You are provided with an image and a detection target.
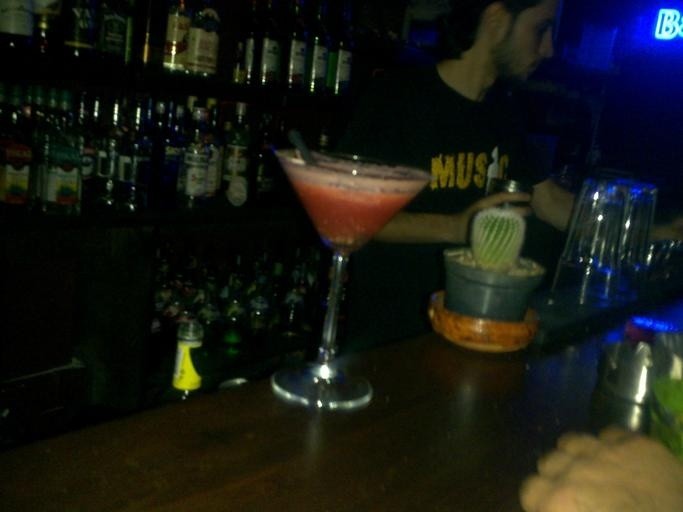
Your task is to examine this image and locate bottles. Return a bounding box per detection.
[158,3,354,97]
[155,247,353,398]
[0,1,151,68]
[2,85,352,219]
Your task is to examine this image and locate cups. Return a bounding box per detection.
[620,180,659,270]
[561,178,624,271]
[487,177,536,214]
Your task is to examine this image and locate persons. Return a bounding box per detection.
[514,422,682,511]
[340,0,682,358]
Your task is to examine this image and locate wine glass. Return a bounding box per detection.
[268,148,431,412]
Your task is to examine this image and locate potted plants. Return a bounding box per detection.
[444,209,546,323]
